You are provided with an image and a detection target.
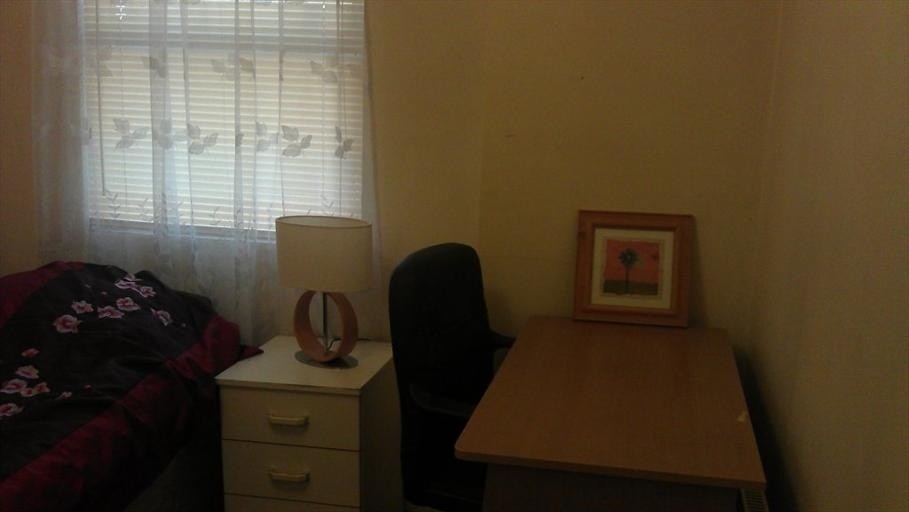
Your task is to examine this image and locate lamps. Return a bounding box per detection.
[276,215,372,363]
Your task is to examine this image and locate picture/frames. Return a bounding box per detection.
[575,210,694,327]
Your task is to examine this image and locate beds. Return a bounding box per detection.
[2,261,223,510]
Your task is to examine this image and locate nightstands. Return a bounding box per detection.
[216,335,403,511]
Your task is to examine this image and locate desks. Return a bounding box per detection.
[455,319,767,512]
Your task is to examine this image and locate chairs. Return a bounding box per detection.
[389,244,519,512]
